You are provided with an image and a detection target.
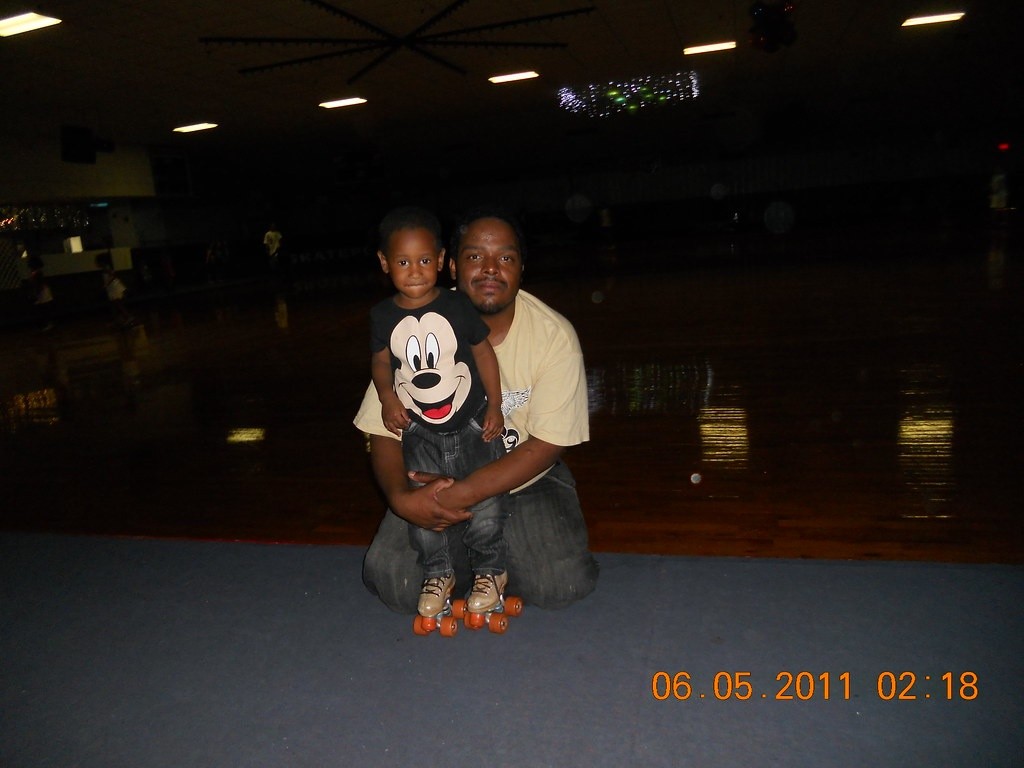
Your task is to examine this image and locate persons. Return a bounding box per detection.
[205,242,226,283]
[263,222,283,255]
[15,242,178,332]
[352,211,600,614]
[598,202,616,251]
[369,207,523,636]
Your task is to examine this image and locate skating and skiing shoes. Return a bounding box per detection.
[464,568,523,634]
[413,571,467,638]
